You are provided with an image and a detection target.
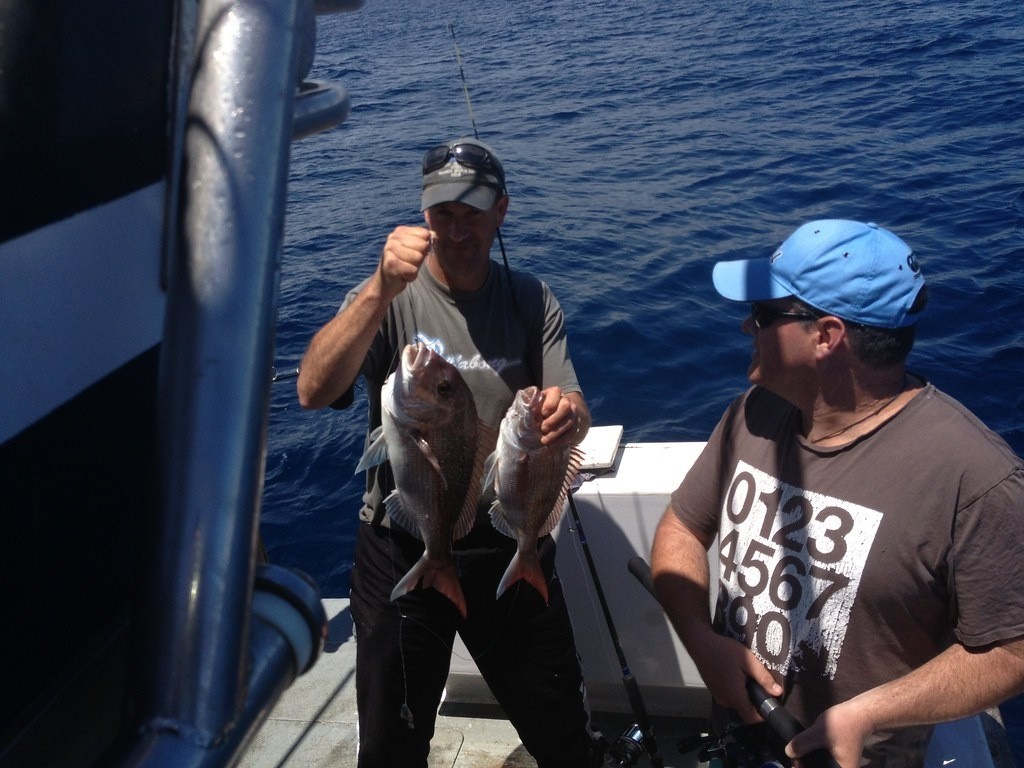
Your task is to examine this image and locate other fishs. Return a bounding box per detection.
[355,341,499,619]
[481,387,587,606]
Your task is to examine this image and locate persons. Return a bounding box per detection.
[647,217,1024,768]
[293,135,615,768]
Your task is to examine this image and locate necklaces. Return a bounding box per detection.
[810,375,907,446]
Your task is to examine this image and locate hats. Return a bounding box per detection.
[421,146,505,214]
[712,218,931,330]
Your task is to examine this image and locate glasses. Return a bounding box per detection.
[422,141,505,184]
[743,301,819,332]
